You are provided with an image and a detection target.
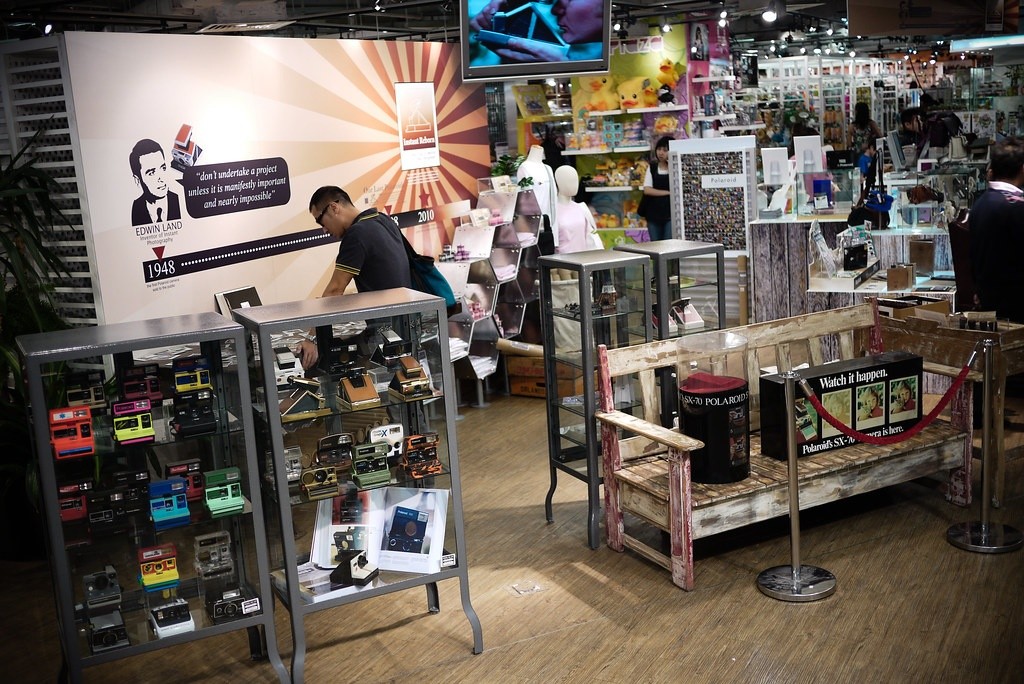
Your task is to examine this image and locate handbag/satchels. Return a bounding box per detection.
[398,226,456,308]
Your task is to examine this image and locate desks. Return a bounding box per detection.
[131,320,363,372]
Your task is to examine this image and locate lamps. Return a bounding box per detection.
[660,13,672,33]
[782,12,965,66]
[719,0,730,29]
[761,0,777,23]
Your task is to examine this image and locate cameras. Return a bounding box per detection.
[46,329,443,653]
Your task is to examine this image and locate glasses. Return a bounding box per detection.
[315,200,339,228]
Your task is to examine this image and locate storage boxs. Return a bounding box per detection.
[505,351,598,399]
[887,263,916,291]
[758,349,923,461]
[603,117,642,149]
[808,259,881,291]
[952,110,996,143]
[878,295,997,329]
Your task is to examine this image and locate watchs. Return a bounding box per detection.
[305,335,317,345]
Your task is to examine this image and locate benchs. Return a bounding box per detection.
[597,303,995,592]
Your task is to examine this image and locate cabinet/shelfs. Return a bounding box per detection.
[691,54,936,148]
[538,249,653,552]
[233,286,483,684]
[750,167,976,313]
[12,310,292,684]
[878,315,1024,508]
[560,105,688,247]
[435,187,543,409]
[614,238,727,438]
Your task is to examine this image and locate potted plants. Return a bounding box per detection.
[1003,64,1024,97]
[0,114,119,576]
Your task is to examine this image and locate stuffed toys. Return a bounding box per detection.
[568,57,680,229]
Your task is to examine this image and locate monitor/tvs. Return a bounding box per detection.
[459,0,612,84]
[886,130,906,170]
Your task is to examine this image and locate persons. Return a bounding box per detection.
[789,127,833,204]
[901,107,923,147]
[857,134,894,177]
[891,380,915,414]
[295,186,435,489]
[968,136,1024,325]
[552,165,589,281]
[468,0,604,62]
[857,387,883,421]
[636,136,681,301]
[517,144,559,247]
[846,102,882,154]
